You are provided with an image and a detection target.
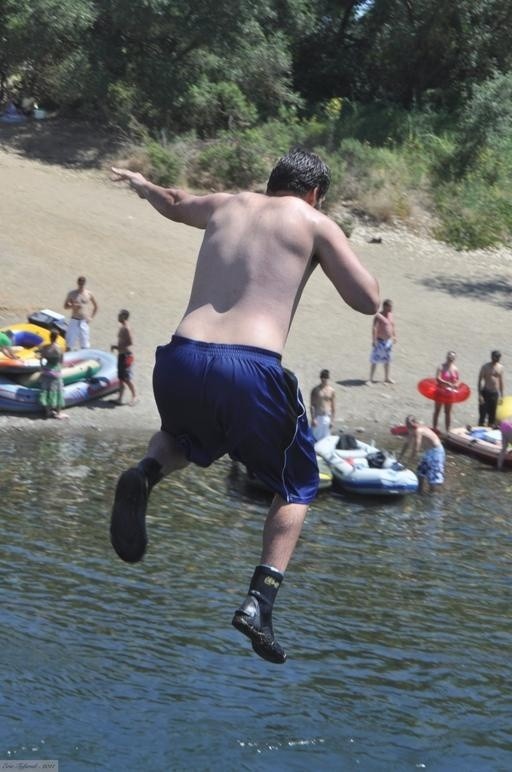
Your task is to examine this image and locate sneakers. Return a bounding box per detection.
[232,592,286,663]
[111,466,149,564]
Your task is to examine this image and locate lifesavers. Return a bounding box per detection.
[418,378,471,403]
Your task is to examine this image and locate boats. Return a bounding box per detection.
[237,435,418,496]
[1,308,69,374]
[1,348,128,416]
[447,424,511,464]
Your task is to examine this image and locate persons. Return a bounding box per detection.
[464,425,501,446]
[370,300,397,383]
[310,370,336,441]
[0,330,20,359]
[497,420,512,469]
[64,277,99,351]
[478,351,505,426]
[109,309,139,406]
[394,415,445,493]
[110,149,381,663]
[433,351,463,431]
[38,329,69,418]
